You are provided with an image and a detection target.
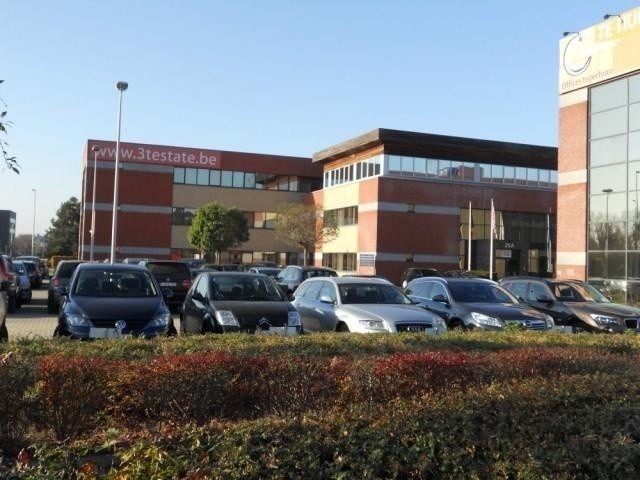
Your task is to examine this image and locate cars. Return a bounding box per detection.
[588,276,639,298]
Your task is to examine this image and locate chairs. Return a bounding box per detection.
[561,288,572,299]
[344,287,382,302]
[83,275,140,294]
[213,280,262,301]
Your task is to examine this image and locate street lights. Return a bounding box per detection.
[111,81,128,263]
[601,187,613,277]
[90,145,101,262]
[32,189,37,255]
